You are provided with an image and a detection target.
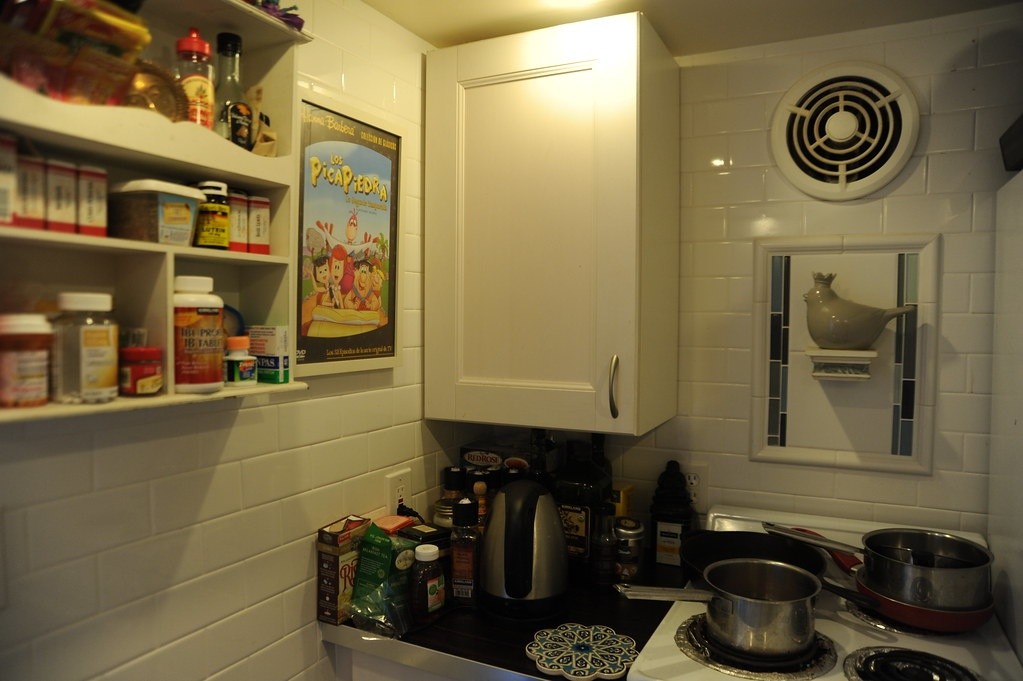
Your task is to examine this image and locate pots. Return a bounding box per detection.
[762,520,995,610]
[788,527,997,634]
[678,528,881,610]
[611,556,822,662]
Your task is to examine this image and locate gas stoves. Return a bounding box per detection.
[626,505,1023,681]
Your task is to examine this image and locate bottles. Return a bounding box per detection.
[224,336,258,387]
[215,32,260,152]
[433,466,488,527]
[0,314,54,410]
[647,461,700,568]
[591,503,618,594]
[48,291,120,406]
[446,497,483,609]
[409,543,447,623]
[193,180,230,251]
[121,346,163,397]
[172,27,216,131]
[172,274,226,395]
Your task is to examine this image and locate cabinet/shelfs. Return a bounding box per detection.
[424,11,681,437]
[0,1,310,425]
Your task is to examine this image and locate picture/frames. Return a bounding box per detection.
[294,83,406,378]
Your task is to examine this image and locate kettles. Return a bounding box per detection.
[476,478,569,624]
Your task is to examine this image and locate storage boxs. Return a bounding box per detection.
[246,324,289,384]
[229,193,270,256]
[0,134,107,238]
[318,513,452,630]
[455,441,533,474]
[999,116,1023,170]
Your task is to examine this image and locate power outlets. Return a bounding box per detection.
[679,465,708,515]
[385,468,412,516]
